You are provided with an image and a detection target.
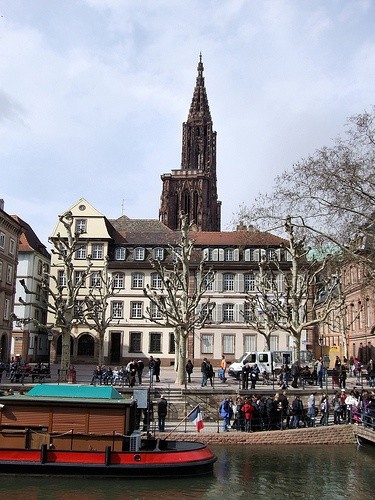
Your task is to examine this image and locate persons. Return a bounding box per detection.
[241,364,260,390]
[200,358,213,387]
[157,395,167,432]
[220,355,227,383]
[90,359,144,388]
[148,356,156,383]
[331,387,375,431]
[308,390,331,426]
[219,390,308,433]
[278,355,326,389]
[186,360,194,383]
[0,355,48,383]
[332,355,375,388]
[154,358,161,382]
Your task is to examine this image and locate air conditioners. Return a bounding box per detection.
[129,429,142,452]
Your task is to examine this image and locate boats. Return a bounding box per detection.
[0,383,218,478]
[354,407,375,448]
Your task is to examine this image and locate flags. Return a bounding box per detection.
[188,406,205,434]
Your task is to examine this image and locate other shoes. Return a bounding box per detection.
[224,430,229,432]
[232,425,307,433]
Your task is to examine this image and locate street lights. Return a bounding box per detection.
[317,335,324,361]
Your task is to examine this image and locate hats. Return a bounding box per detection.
[270,395,274,397]
[253,364,257,367]
[161,395,164,399]
[296,396,300,398]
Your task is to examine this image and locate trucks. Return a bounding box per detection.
[227,351,314,381]
